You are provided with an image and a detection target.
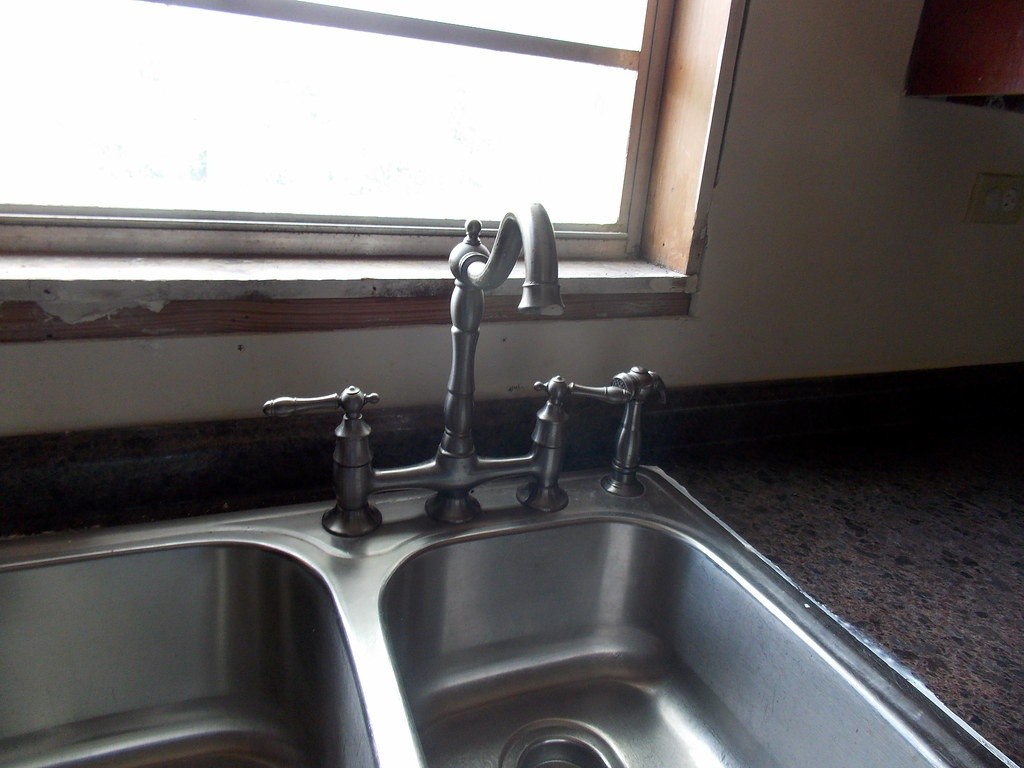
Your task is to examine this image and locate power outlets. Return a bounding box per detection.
[961,173,1024,225]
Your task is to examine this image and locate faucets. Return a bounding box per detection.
[600,366,672,498]
[262,202,633,540]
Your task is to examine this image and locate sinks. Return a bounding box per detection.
[337,460,1022,768]
[0,494,406,768]
